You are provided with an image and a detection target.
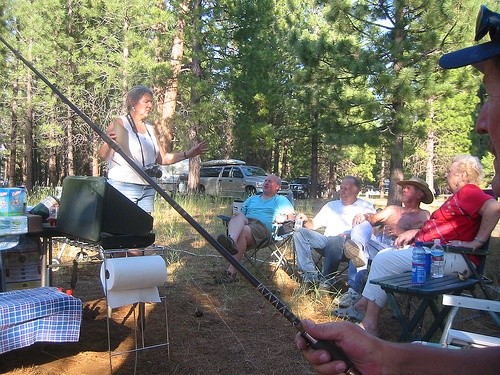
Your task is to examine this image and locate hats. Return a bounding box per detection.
[439,5,500,69]
[396,176,434,204]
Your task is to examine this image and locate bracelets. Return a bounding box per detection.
[474,236,485,244]
[184,151,188,158]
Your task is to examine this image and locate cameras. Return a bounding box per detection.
[142,164,163,178]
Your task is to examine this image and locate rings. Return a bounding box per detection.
[458,241,461,245]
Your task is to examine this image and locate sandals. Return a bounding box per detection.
[333,305,365,321]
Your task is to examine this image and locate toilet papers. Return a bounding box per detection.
[99,255,168,308]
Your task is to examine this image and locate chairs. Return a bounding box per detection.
[368,189,498,339]
[289,208,382,299]
[412,294,500,350]
[217,190,305,282]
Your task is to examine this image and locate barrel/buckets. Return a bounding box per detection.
[232,199,245,215]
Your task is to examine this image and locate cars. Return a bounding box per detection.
[289,176,327,200]
[156,166,186,193]
[359,184,390,197]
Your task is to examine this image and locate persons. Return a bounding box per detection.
[334,153,499,339]
[97,87,208,283]
[294,175,378,299]
[292,5,500,375]
[333,177,434,308]
[213,173,296,285]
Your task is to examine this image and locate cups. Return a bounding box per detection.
[49,207,58,226]
[382,226,392,245]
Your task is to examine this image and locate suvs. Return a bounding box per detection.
[199,159,291,203]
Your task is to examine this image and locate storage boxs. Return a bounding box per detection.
[0,187,44,235]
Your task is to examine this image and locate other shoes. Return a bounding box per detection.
[217,234,239,255]
[304,281,319,295]
[222,271,237,283]
[335,288,361,308]
[317,286,332,299]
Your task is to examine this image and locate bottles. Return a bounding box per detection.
[412,240,427,287]
[430,238,444,279]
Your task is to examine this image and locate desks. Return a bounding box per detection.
[0,286,83,360]
[369,272,481,343]
[41,232,170,375]
